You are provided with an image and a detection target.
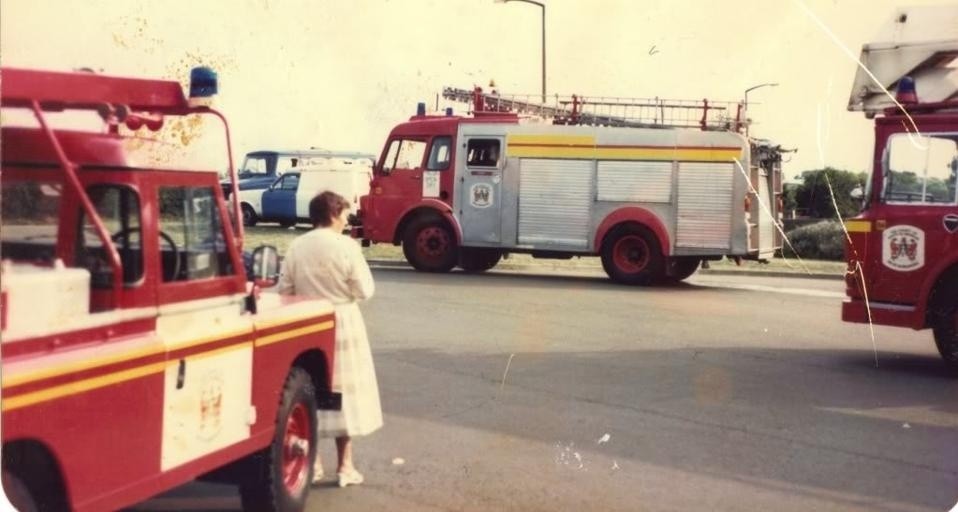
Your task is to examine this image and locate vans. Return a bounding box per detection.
[229,168,375,228]
[217,150,376,202]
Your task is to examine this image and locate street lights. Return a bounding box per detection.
[496,0,548,104]
[744,83,777,137]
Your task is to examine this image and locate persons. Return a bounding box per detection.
[275,191,384,488]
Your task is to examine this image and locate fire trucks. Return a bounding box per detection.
[839,38,958,376]
[350,83,788,288]
[0,61,347,511]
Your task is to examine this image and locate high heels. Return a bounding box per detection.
[312,465,324,484]
[336,468,363,487]
